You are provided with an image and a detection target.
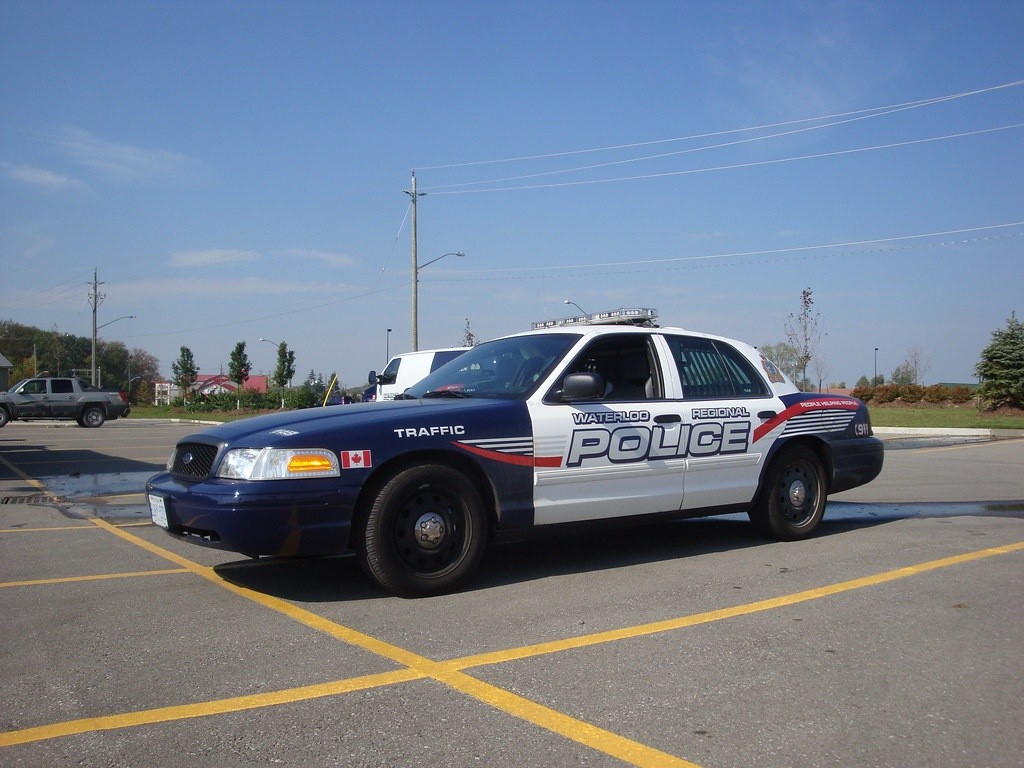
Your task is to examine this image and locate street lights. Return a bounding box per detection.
[92,316,136,386]
[412,252,465,352]
[35,371,48,391]
[386,329,392,364]
[875,348,879,387]
[260,339,291,389]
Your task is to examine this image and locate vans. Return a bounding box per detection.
[362,347,497,402]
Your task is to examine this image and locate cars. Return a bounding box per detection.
[0,378,131,428]
[314,395,355,407]
[145,308,886,599]
